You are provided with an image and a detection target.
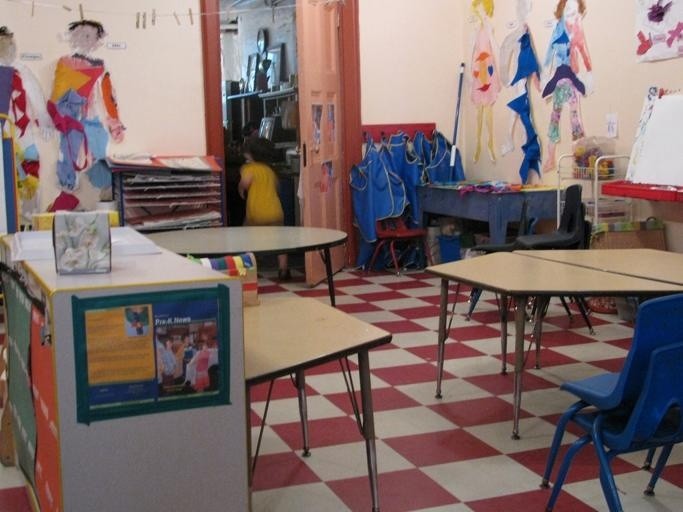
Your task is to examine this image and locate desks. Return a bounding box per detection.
[140,223,350,304]
[426,238,683,439]
[416,180,563,249]
[240,286,394,510]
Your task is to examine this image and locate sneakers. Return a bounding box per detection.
[277,267,291,282]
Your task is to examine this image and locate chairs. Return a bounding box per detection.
[538,292,683,512]
[366,208,433,276]
[466,184,602,340]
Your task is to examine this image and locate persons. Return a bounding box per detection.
[156,322,221,395]
[234,135,294,281]
